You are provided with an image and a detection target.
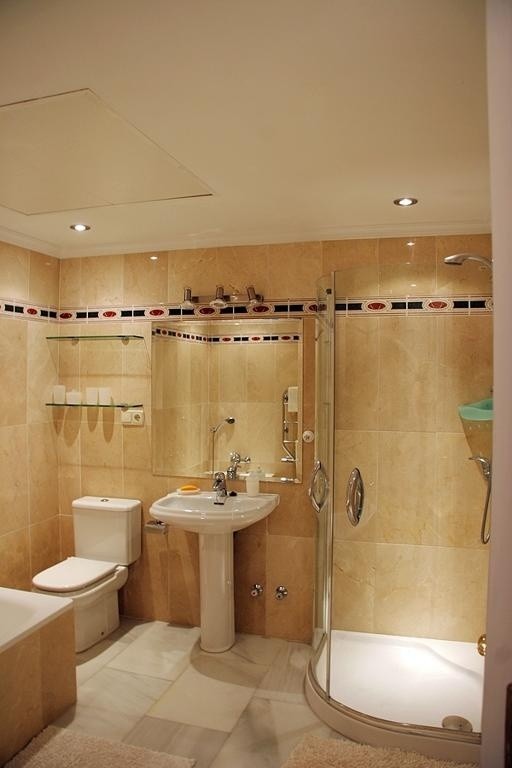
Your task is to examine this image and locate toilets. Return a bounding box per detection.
[29,495,143,655]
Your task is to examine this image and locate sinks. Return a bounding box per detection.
[148,490,280,535]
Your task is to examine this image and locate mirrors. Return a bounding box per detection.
[149,316,303,485]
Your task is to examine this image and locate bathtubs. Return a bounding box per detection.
[0,587,74,655]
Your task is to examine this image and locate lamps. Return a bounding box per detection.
[177,283,263,311]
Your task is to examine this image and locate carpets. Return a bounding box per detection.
[279,731,478,768]
[4,724,198,768]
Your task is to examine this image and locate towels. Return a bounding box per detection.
[287,386,298,414]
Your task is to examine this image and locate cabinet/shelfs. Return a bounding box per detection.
[46,334,144,408]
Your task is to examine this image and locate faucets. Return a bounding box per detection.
[226,451,240,480]
[212,472,228,505]
